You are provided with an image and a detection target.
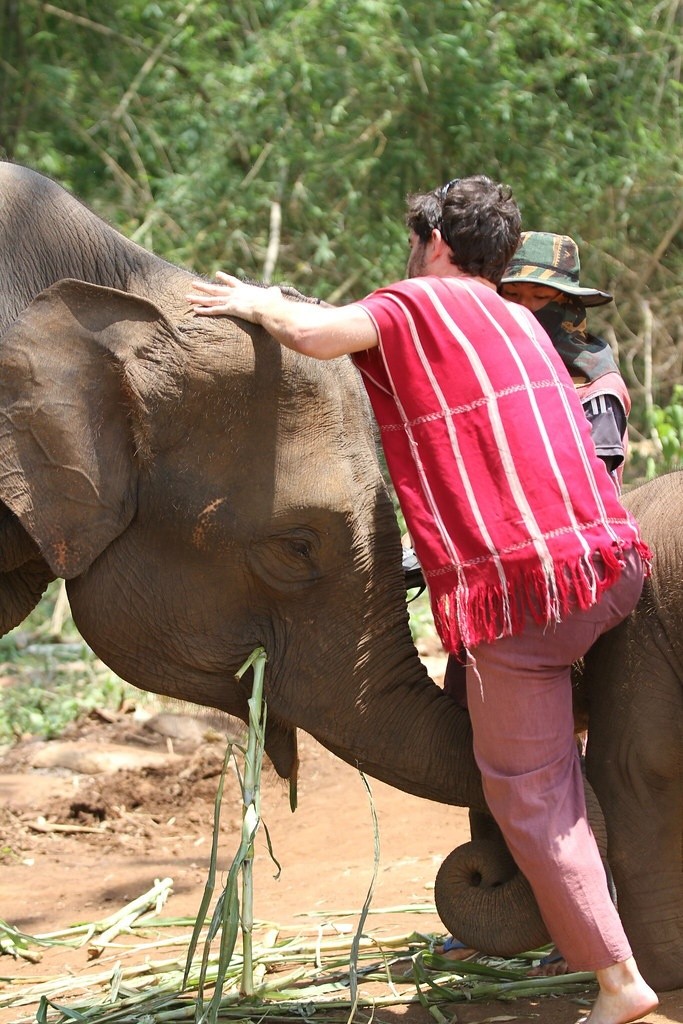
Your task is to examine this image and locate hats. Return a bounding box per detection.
[499,232,613,307]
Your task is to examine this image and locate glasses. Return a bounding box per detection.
[440,178,460,241]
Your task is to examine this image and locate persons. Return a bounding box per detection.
[398,231,631,982]
[188,171,661,1023]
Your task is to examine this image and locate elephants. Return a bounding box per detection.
[443,466,683,990]
[0,159,610,958]
